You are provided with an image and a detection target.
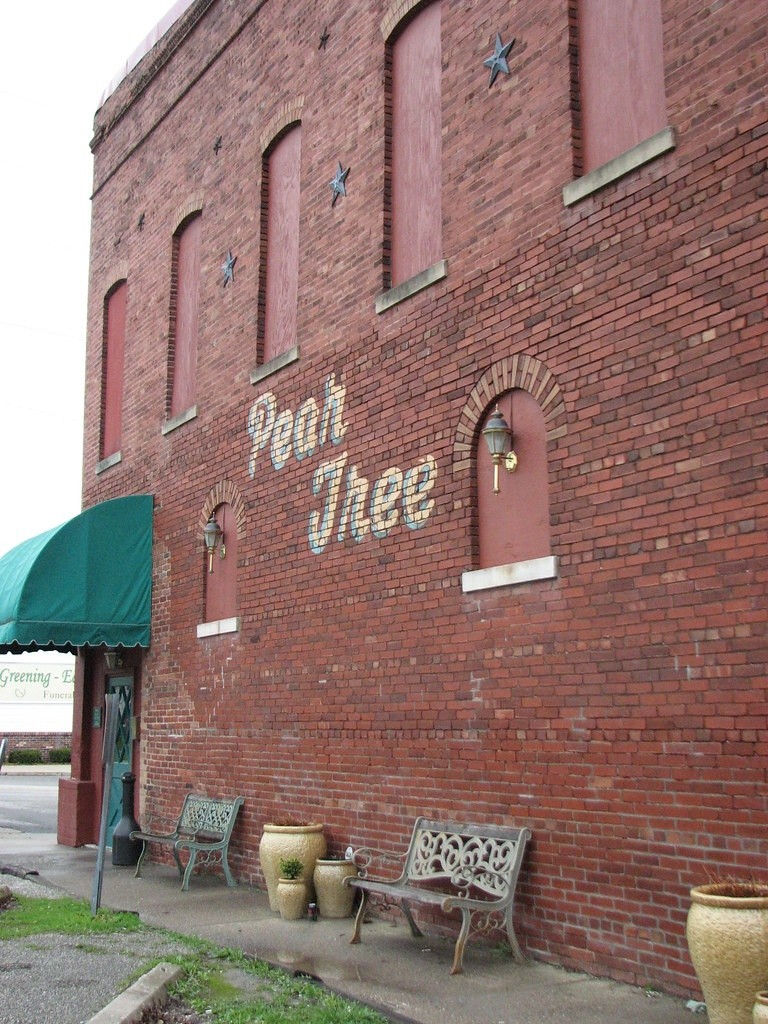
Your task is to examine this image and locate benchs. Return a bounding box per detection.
[128,792,244,893]
[343,815,532,975]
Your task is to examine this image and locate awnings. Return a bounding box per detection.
[0,494,154,654]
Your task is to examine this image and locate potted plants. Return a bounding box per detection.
[277,856,309,920]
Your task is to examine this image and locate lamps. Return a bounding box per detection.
[202,511,226,576]
[481,403,518,495]
